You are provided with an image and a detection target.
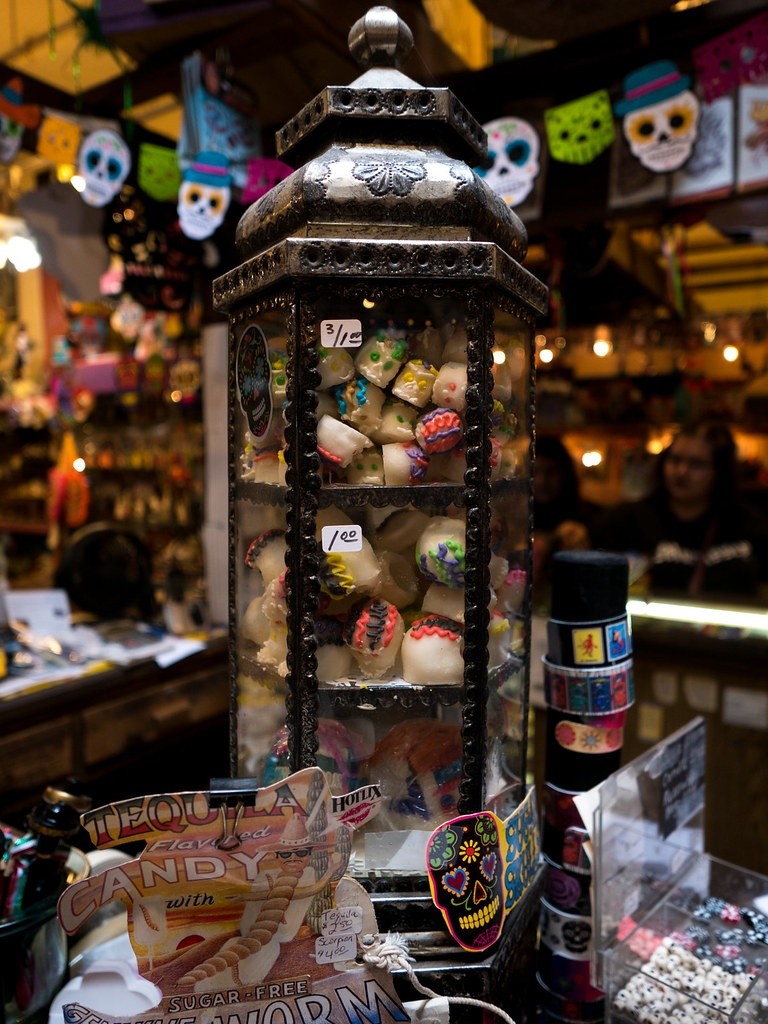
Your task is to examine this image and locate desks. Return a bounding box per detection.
[0,611,232,827]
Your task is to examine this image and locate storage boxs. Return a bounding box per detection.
[594,854,768,1024]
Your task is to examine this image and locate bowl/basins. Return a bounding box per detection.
[0,874,70,1024]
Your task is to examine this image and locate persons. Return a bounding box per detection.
[53,483,165,624]
[531,436,590,588]
[595,420,768,598]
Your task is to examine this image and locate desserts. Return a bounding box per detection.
[229,308,529,829]
